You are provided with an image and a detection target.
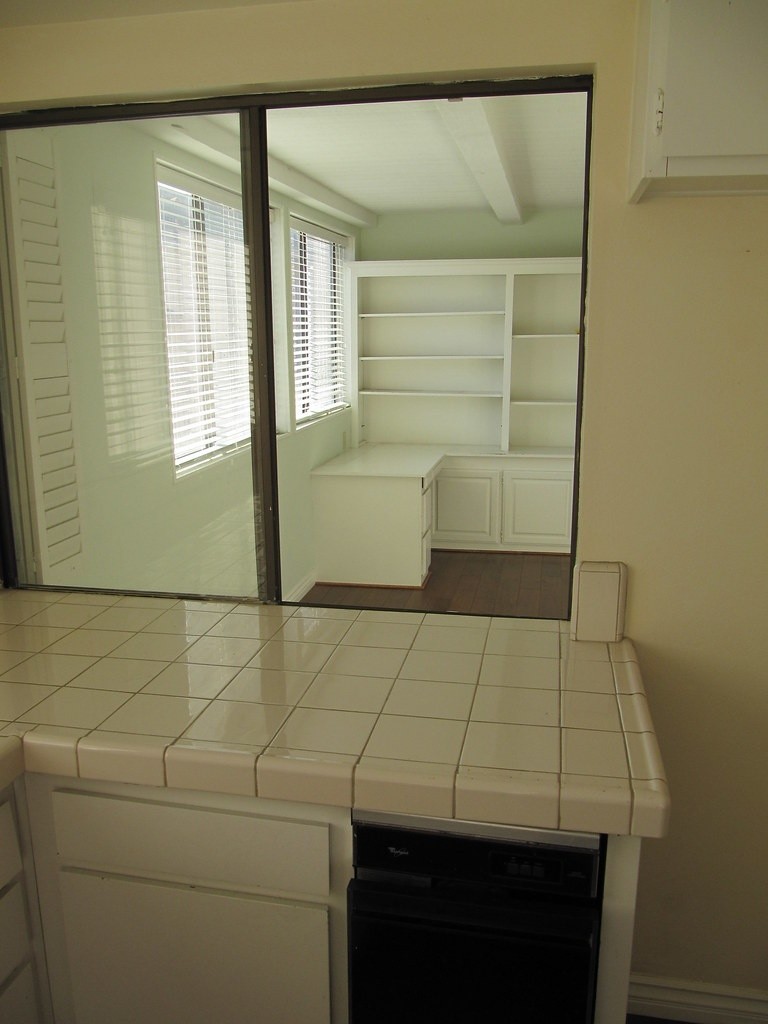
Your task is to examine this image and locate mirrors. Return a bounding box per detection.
[0,88,590,620]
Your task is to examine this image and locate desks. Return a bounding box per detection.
[312,448,574,589]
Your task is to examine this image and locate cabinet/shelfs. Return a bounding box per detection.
[0,584,669,1024]
[350,256,582,453]
[625,0,768,204]
